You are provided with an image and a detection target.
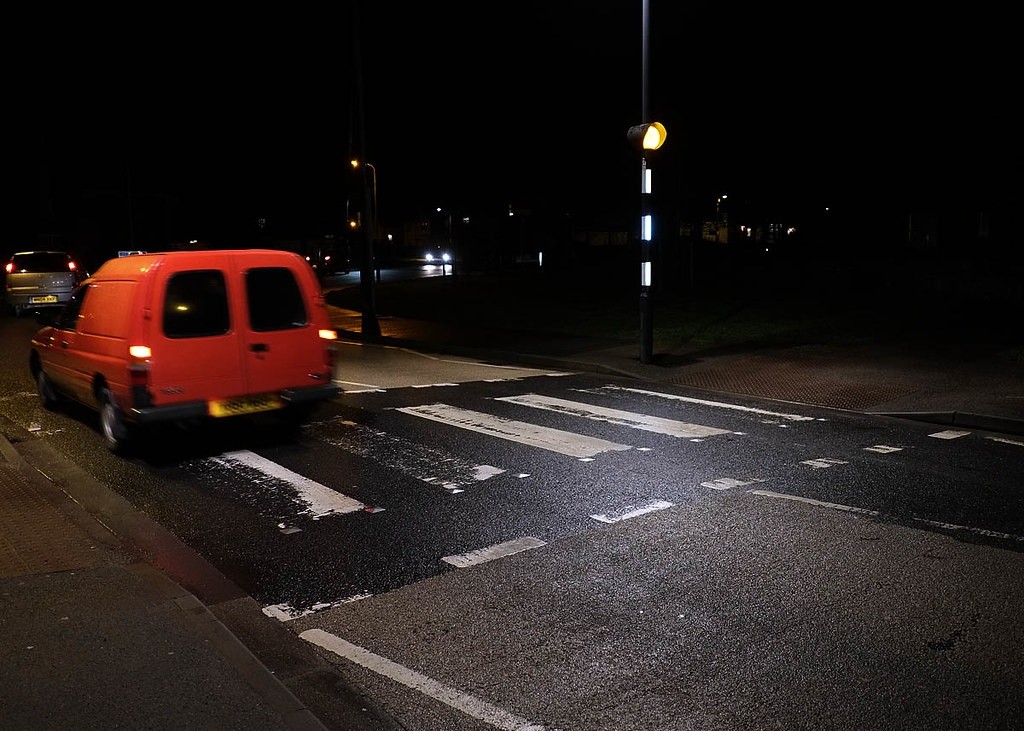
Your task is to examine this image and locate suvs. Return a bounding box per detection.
[6,252,87,315]
[30,249,339,453]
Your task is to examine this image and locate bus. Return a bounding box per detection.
[318,235,376,274]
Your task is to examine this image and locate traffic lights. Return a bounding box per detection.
[349,211,361,228]
[625,121,668,153]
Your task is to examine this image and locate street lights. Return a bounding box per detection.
[350,160,379,230]
[508,204,514,250]
[825,206,829,239]
[715,193,727,219]
[436,207,451,227]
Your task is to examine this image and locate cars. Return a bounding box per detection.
[422,245,456,266]
